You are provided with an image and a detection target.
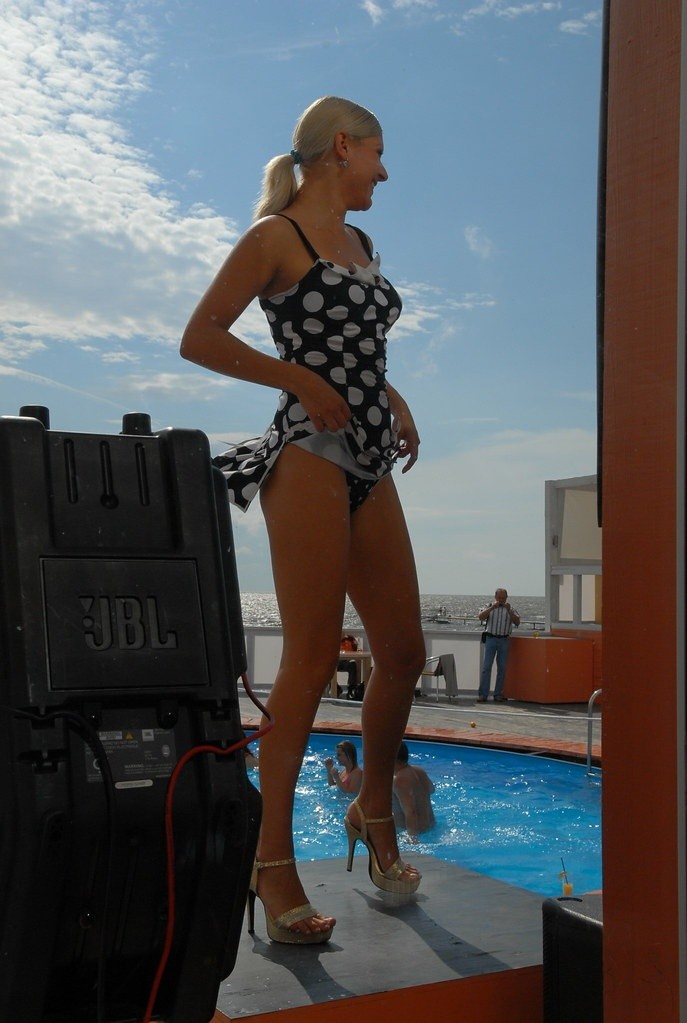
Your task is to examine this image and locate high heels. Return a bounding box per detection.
[343,796,421,894]
[246,857,333,944]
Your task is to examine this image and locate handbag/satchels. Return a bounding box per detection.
[482,631,487,643]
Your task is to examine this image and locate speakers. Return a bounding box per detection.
[542,893,604,1023]
[0,404,265,1023]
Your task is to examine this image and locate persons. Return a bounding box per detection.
[241,731,258,768]
[477,588,520,702]
[323,740,434,842]
[327,631,362,699]
[179,96,435,935]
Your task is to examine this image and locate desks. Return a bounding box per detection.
[330,651,373,698]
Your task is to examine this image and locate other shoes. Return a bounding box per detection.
[477,695,487,702]
[337,687,342,697]
[494,695,507,702]
[347,693,355,699]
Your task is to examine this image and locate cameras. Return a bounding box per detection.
[499,603,505,607]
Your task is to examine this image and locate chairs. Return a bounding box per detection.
[416,653,454,702]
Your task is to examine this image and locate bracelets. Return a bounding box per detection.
[489,605,494,610]
[507,608,510,612]
